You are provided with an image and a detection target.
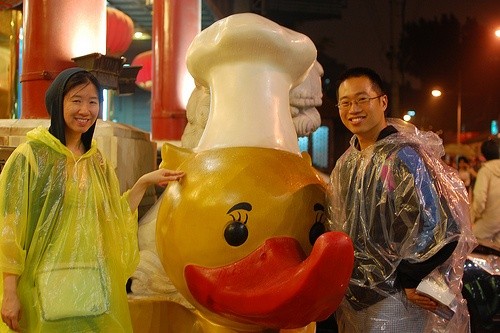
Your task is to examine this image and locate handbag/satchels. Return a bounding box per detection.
[34,261,107,323]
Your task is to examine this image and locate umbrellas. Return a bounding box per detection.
[445,144,475,171]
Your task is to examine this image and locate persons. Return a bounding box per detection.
[0,69,186,333]
[325,67,481,333]
[444,135,500,333]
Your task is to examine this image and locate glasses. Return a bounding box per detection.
[336,95,384,110]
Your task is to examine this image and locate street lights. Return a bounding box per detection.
[431,87,461,146]
[404,115,412,121]
[495,27,500,38]
